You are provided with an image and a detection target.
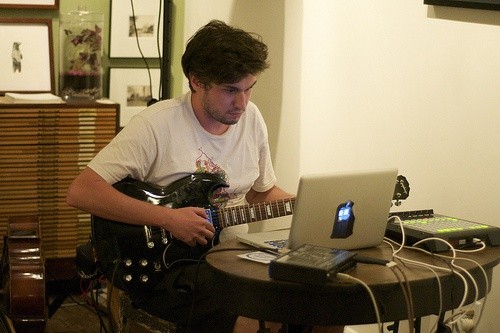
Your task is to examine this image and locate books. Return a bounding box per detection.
[5,92,62,103]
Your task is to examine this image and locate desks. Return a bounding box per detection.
[206,227,500,333]
[0,95,121,267]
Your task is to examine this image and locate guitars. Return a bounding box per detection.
[92,173,411,296]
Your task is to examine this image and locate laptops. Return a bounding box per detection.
[236,168,398,256]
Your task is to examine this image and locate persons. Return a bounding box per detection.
[66,20,345,333]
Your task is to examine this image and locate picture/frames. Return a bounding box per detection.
[0,18,56,95]
[0,0,59,10]
